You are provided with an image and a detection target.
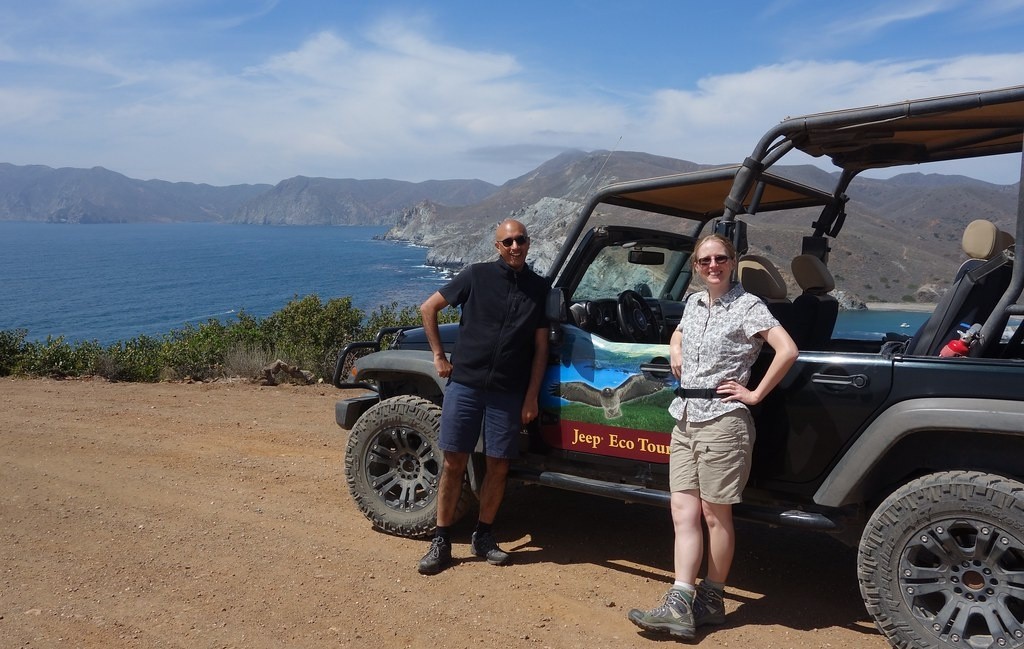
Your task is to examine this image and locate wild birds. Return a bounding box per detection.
[548,374,671,421]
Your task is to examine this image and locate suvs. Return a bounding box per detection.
[332,85,1024,648]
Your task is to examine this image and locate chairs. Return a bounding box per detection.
[792,253,840,348]
[738,253,795,341]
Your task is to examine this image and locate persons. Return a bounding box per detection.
[627,233,799,640]
[418,219,551,574]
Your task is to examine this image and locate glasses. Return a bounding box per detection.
[696,255,734,265]
[497,235,527,247]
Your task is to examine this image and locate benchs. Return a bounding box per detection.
[897,220,1014,357]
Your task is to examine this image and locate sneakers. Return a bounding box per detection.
[418,536,452,575]
[471,531,509,565]
[691,580,725,626]
[628,587,697,642]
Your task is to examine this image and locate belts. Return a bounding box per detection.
[674,386,734,399]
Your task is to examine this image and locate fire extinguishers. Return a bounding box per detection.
[939,324,984,358]
